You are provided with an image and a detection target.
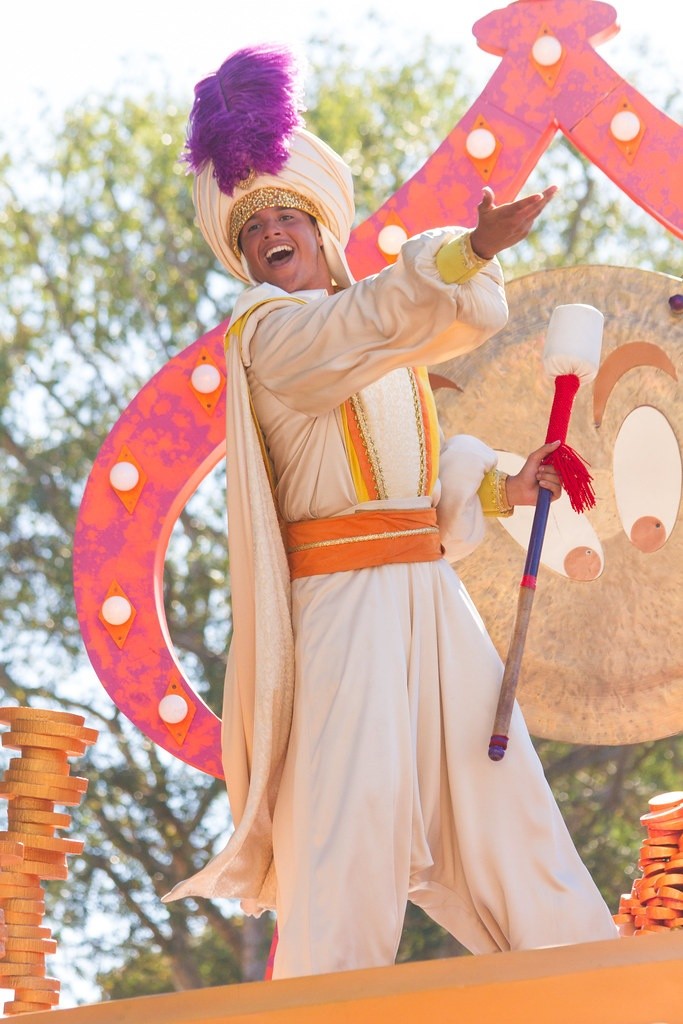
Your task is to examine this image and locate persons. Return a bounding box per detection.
[158,122,626,977]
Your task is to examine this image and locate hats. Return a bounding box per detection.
[180,43,357,284]
